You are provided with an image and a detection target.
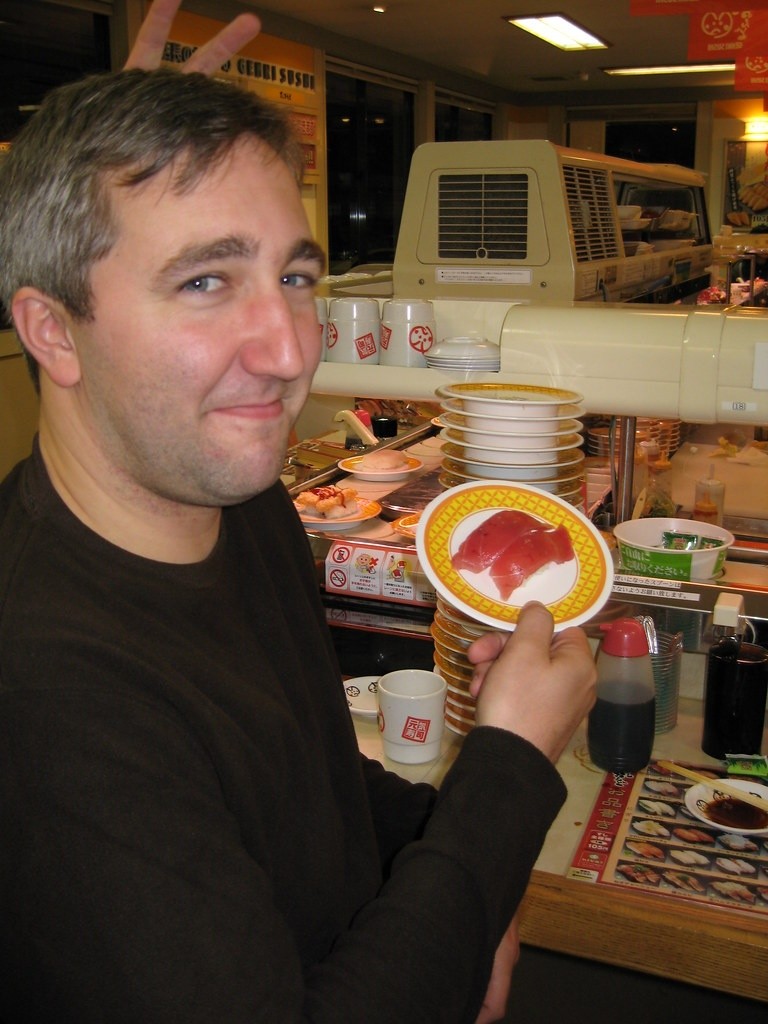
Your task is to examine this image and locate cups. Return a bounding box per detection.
[314,295,328,362]
[327,297,380,364]
[377,669,448,765]
[632,630,684,735]
[701,642,768,760]
[379,298,437,366]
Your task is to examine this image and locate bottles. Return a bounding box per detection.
[692,461,726,528]
[587,618,657,775]
[344,409,375,450]
[633,429,673,495]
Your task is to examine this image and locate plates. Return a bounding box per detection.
[619,219,652,230]
[338,457,425,482]
[343,676,382,718]
[429,589,511,738]
[415,479,615,634]
[292,497,382,531]
[423,336,500,372]
[684,779,768,835]
[391,513,421,539]
[588,416,681,457]
[431,382,586,509]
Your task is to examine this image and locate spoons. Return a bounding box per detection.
[631,614,684,653]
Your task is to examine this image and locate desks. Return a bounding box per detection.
[343,673,768,1004]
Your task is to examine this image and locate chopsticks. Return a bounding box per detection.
[657,759,768,812]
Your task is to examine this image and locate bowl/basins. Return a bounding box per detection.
[612,517,735,582]
[618,205,642,219]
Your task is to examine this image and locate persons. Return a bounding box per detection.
[732,224,768,308]
[0,0,605,1024]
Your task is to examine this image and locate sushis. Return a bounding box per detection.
[297,486,341,515]
[317,489,358,518]
